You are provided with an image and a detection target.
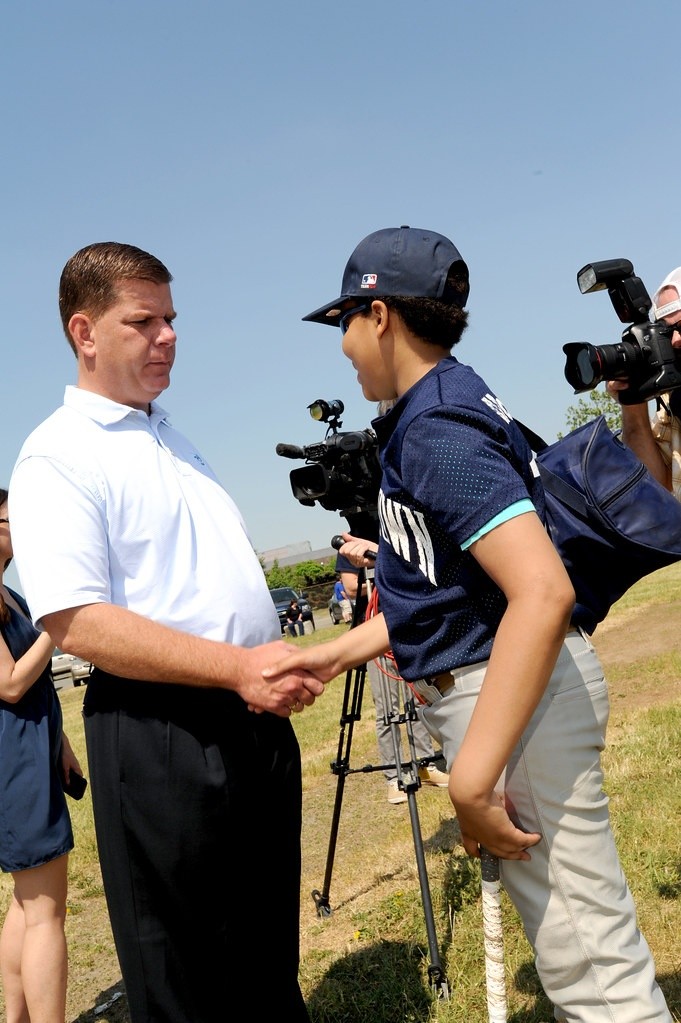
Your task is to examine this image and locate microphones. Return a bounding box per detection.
[331,535,378,560]
[275,443,305,460]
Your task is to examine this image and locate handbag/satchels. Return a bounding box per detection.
[515,418,680,627]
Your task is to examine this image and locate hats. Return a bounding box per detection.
[302,226,470,327]
[651,267,681,322]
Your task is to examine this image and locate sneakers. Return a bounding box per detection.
[388,783,408,803]
[417,766,449,789]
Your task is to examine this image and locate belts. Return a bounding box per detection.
[418,624,577,707]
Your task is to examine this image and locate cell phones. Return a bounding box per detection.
[62,769,86,801]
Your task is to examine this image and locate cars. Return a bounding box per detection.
[68,656,92,687]
[51,645,74,676]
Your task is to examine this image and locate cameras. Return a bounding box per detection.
[561,258,681,406]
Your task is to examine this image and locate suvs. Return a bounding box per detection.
[269,587,317,633]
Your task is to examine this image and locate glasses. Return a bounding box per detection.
[668,320,681,340]
[340,300,370,336]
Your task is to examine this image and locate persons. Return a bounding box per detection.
[8,243,325,1022]
[605,263,681,494]
[246,224,676,1023]
[1,489,85,1022]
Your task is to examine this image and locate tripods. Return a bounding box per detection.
[313,504,450,998]
